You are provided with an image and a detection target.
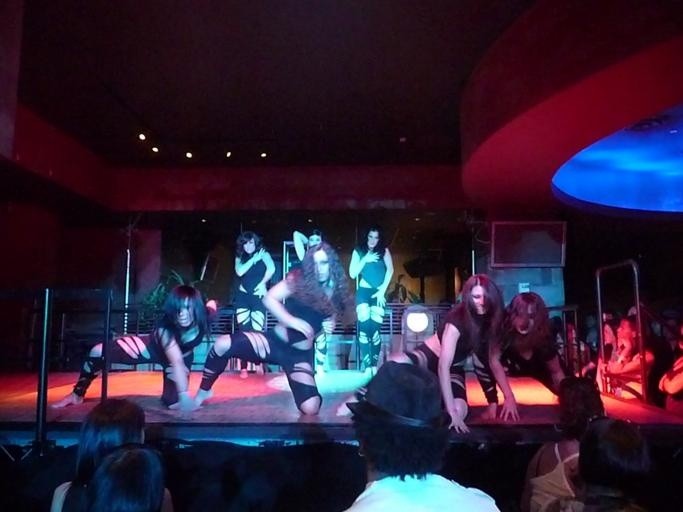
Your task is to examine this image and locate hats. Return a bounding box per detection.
[346,360,452,433]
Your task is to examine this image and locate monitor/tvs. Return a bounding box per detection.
[490,221,567,268]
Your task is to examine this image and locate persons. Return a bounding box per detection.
[335,272,520,434]
[291,228,334,380]
[565,295,682,425]
[193,242,361,416]
[48,359,657,511]
[472,291,575,423]
[48,284,214,422]
[232,231,277,378]
[347,228,391,381]
